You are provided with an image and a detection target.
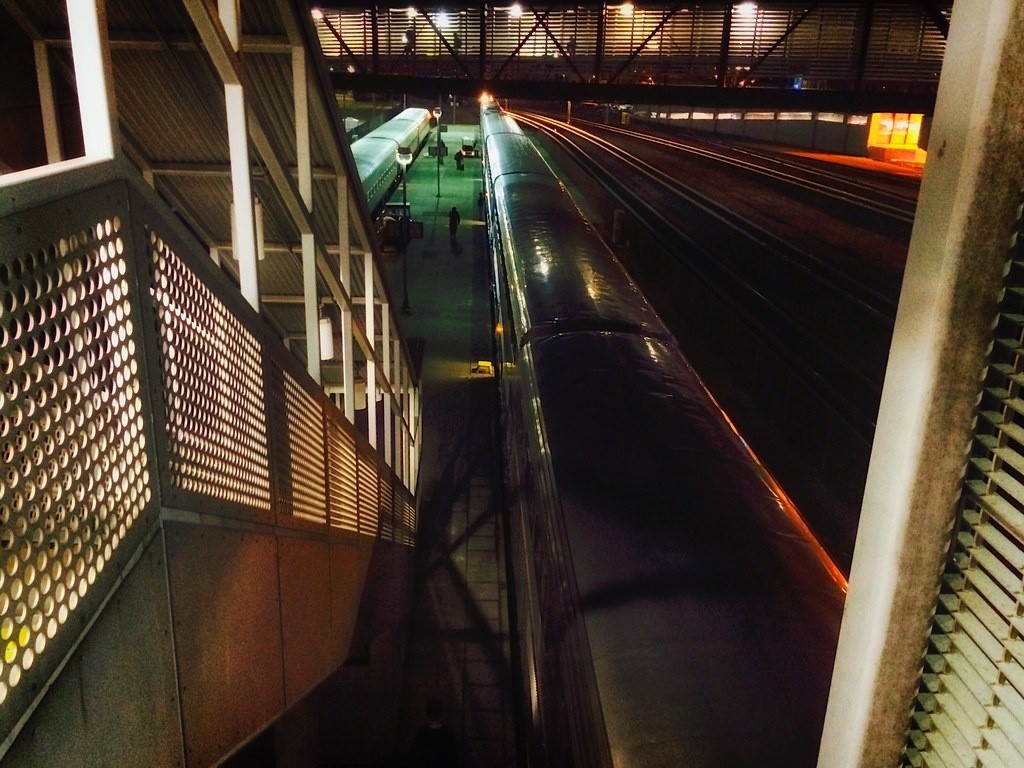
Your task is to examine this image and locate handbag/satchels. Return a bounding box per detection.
[454,156,457,160]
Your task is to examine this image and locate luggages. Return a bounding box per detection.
[460,160,464,170]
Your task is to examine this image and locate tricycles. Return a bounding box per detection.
[378,203,424,251]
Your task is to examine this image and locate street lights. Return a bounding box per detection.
[451,91,459,125]
[433,108,443,199]
[396,146,413,313]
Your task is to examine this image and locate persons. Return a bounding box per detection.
[478,192,484,222]
[449,207,460,239]
[454,150,463,169]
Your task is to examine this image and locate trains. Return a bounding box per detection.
[349,108,433,215]
[482,91,848,767]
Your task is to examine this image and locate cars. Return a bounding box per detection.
[460,144,476,156]
